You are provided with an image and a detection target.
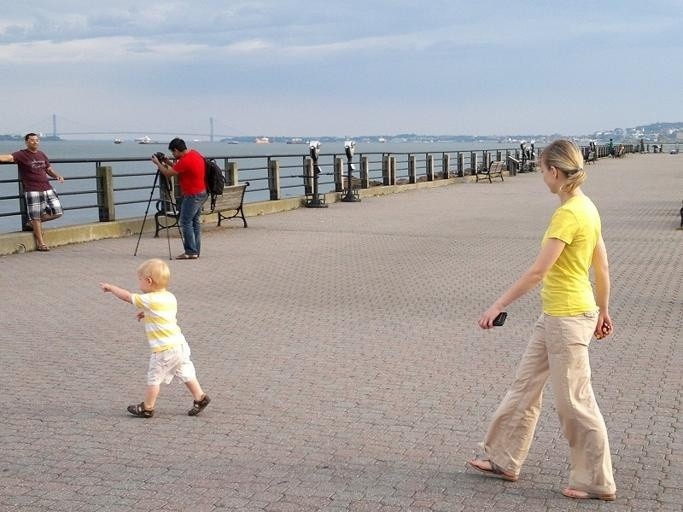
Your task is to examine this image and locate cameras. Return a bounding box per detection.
[152,152,164,160]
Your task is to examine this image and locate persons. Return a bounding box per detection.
[0,133,64,251]
[100,259,210,417]
[639,139,645,151]
[608,139,624,159]
[151,139,208,259]
[468,140,617,501]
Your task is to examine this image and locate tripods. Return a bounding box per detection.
[134,165,186,260]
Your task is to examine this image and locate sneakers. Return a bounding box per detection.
[175,253,198,259]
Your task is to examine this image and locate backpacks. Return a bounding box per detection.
[204,157,226,196]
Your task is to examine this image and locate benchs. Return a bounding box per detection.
[153,181,251,238]
[474,160,506,184]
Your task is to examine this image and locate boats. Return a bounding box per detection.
[227,141,238,144]
[113,139,122,144]
[287,136,306,146]
[255,138,271,145]
[134,137,151,144]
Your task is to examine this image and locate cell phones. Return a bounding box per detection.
[487,313,507,326]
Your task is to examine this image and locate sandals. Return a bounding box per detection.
[468,458,518,483]
[127,401,154,418]
[188,393,211,416]
[37,243,50,251]
[561,486,616,502]
